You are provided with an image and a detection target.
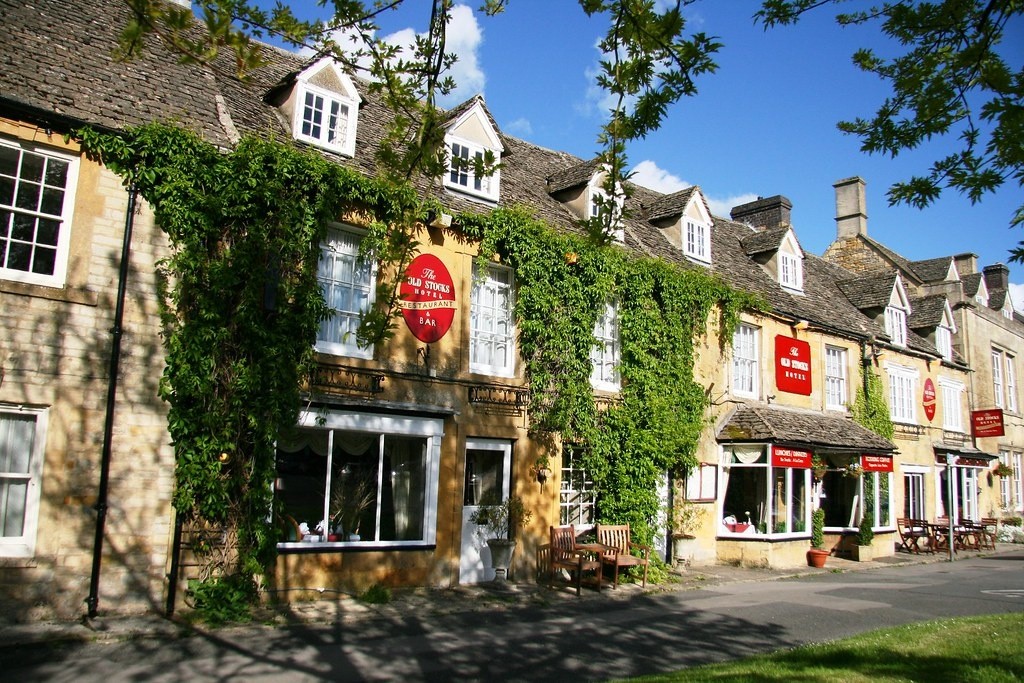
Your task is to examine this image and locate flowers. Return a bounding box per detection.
[327,515,336,525]
[811,454,829,470]
[841,456,862,473]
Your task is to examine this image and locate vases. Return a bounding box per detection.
[849,471,859,478]
[814,469,827,479]
[328,525,333,534]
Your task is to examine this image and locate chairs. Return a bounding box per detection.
[897,515,997,555]
[597,522,650,588]
[550,524,605,596]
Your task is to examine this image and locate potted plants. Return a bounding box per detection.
[808,507,830,567]
[850,512,874,562]
[994,462,1013,477]
[468,496,532,570]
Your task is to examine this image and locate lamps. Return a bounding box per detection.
[875,353,887,360]
[538,466,552,482]
[792,319,808,330]
[926,358,941,366]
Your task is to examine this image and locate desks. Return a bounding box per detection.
[927,523,962,554]
